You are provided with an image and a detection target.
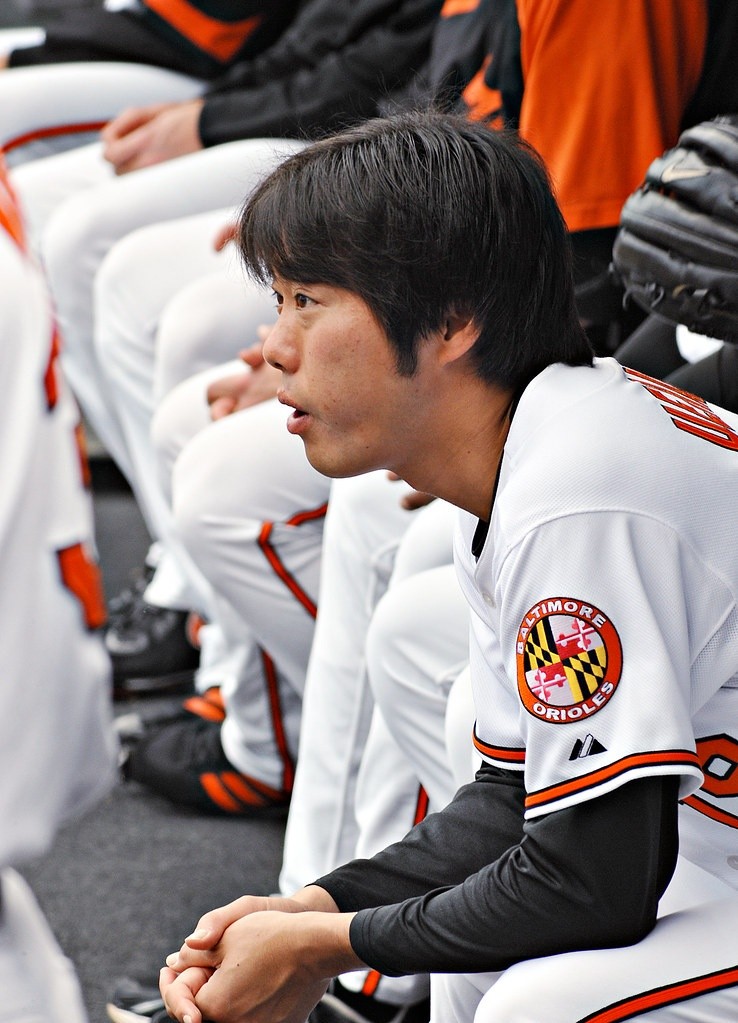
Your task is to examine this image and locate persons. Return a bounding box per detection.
[0,0,738,1023]
[158,114,738,1023]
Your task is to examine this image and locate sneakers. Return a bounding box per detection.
[113,686,291,814]
[100,565,206,695]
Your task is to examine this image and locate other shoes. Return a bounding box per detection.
[106,971,175,1023]
